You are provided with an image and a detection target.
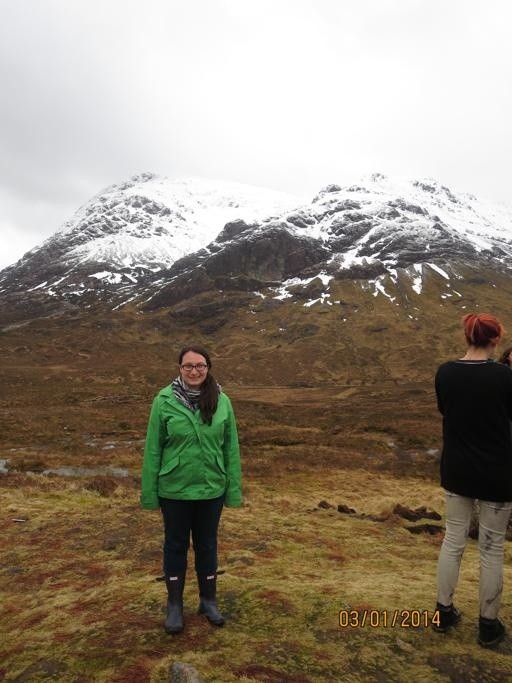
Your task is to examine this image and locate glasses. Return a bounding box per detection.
[182,364,206,371]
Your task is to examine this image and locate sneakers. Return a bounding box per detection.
[479,616,504,646]
[432,602,461,632]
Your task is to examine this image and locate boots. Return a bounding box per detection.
[197,573,224,626]
[165,573,184,634]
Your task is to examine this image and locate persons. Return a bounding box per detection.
[432,311,511,649]
[140,347,242,633]
[496,345,511,368]
